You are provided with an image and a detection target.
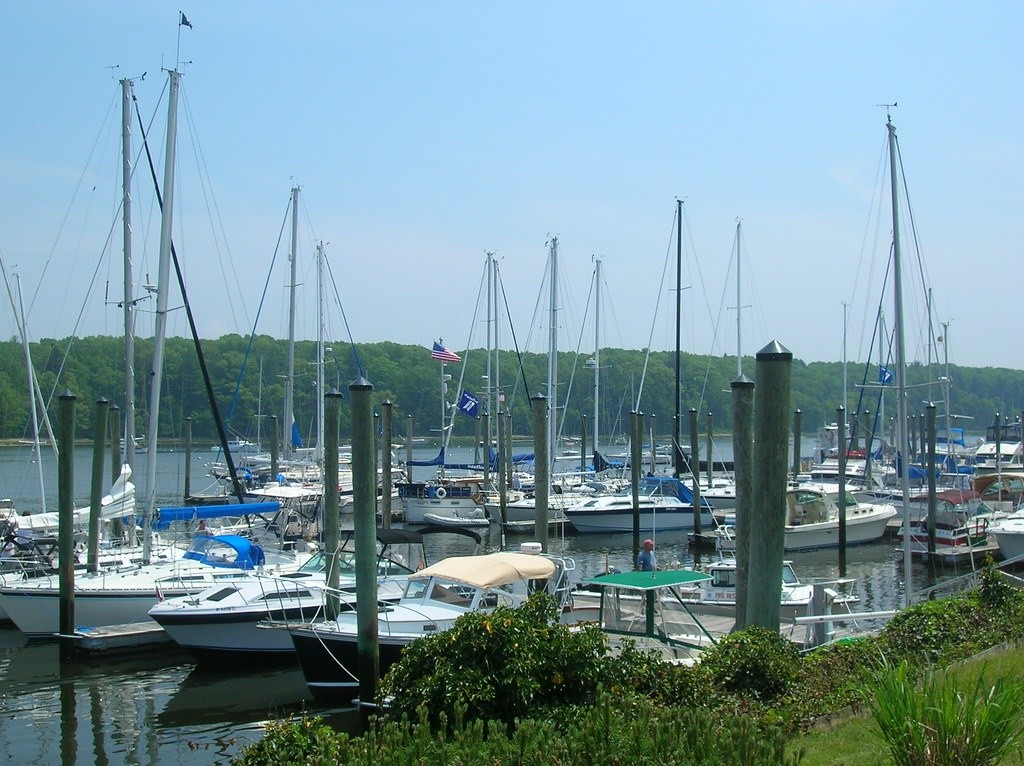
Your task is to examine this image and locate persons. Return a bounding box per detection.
[193,520,214,553]
[637,540,662,615]
[788,492,798,520]
[513,469,525,490]
[0,510,44,558]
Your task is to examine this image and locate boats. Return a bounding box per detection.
[1,632,191,702]
[138,650,313,730]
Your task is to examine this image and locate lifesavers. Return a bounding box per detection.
[436,488,447,499]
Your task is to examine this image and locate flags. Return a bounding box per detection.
[182,12,193,30]
[879,363,895,384]
[292,414,302,448]
[458,390,478,418]
[431,340,461,362]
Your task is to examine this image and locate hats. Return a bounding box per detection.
[644,539,654,545]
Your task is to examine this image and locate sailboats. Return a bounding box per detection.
[0,56,1023,714]
